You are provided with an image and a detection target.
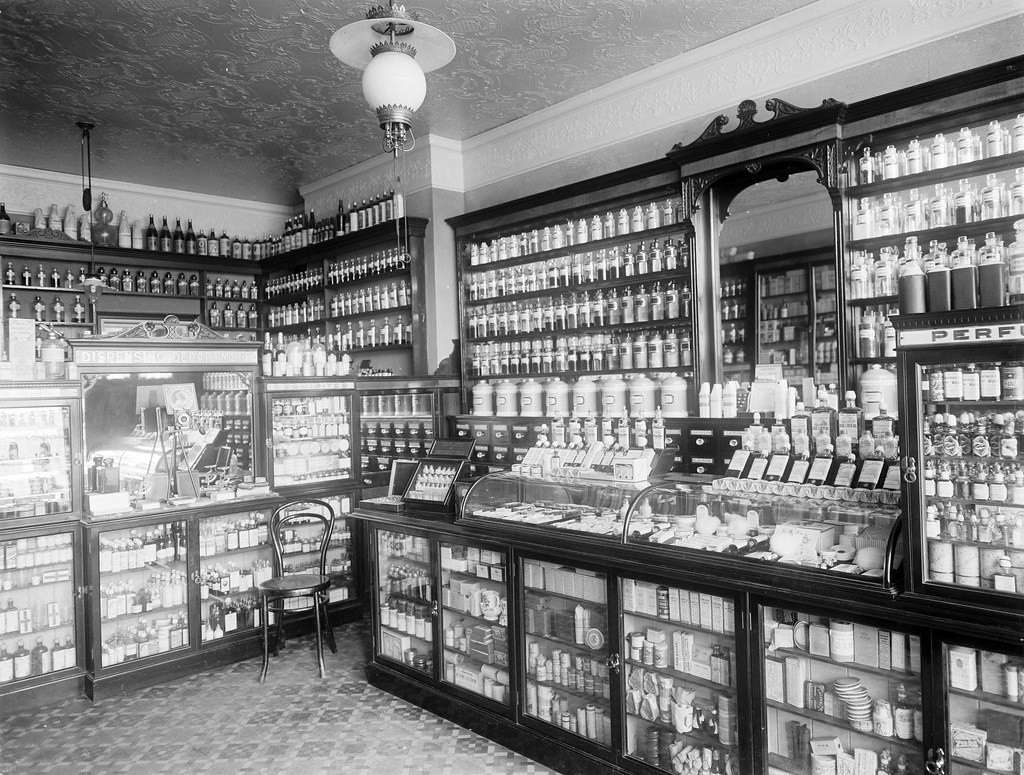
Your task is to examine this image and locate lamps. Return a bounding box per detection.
[77,119,112,304]
[329,0,456,270]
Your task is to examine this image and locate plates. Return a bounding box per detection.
[833,675,872,722]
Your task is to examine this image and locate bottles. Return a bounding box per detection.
[199,371,249,417]
[924,411,1021,459]
[855,109,1024,357]
[377,528,741,774]
[0,174,418,377]
[720,266,837,396]
[927,505,1024,549]
[0,495,355,682]
[696,381,902,491]
[993,559,1017,593]
[271,396,351,479]
[97,458,121,493]
[922,362,1023,400]
[468,373,690,482]
[925,460,1024,503]
[877,683,921,775]
[466,193,695,376]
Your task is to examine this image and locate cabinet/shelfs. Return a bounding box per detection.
[198,373,253,470]
[262,216,429,375]
[0,228,262,360]
[888,304,1024,612]
[85,502,279,677]
[360,375,462,491]
[445,415,898,476]
[0,521,84,716]
[750,592,1024,775]
[444,53,1024,414]
[277,493,361,616]
[720,246,839,386]
[514,548,750,774]
[0,381,83,529]
[369,522,514,722]
[258,375,362,491]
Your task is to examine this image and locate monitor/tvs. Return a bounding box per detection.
[388,460,418,497]
[214,446,232,475]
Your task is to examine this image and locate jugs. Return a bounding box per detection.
[479,590,502,621]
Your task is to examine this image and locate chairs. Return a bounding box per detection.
[258,499,338,683]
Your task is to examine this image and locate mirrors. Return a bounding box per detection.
[76,366,260,522]
[682,137,851,411]
